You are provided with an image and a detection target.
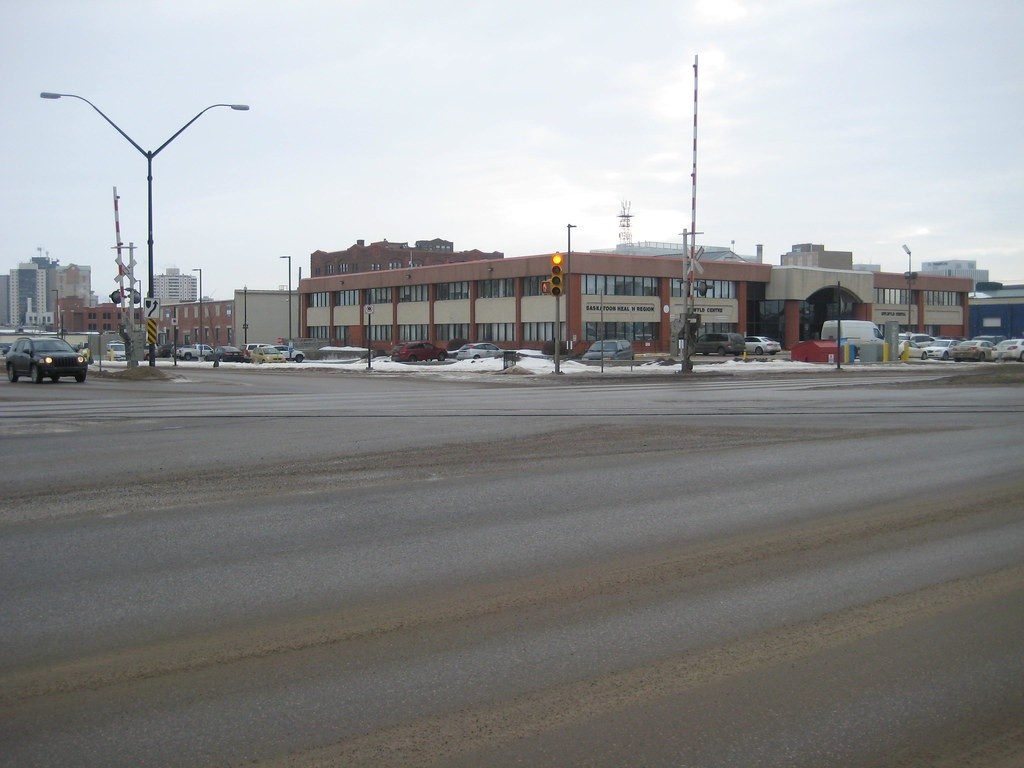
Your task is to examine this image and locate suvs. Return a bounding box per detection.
[6,336,88,384]
[695,333,745,356]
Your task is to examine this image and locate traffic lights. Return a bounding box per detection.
[550,254,563,297]
[542,280,551,294]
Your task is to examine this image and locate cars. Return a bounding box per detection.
[457,343,504,361]
[391,342,447,363]
[0,342,13,355]
[743,336,781,354]
[204,345,306,362]
[105,341,213,361]
[582,339,635,360]
[898,333,1024,362]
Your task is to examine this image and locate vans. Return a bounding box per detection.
[821,320,883,340]
[240,344,274,363]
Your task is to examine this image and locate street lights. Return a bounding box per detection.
[192,268,202,356]
[280,256,291,361]
[903,244,912,337]
[52,289,59,338]
[566,223,577,359]
[41,92,250,367]
